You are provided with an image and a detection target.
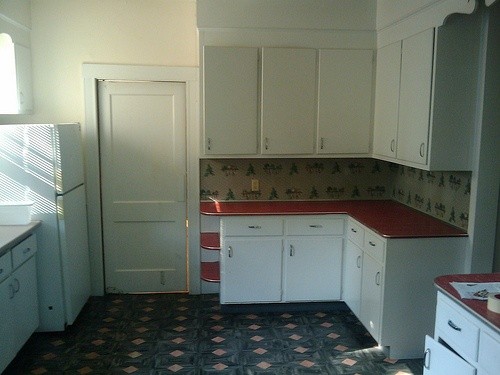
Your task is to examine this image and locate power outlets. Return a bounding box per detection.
[252,179,259,191]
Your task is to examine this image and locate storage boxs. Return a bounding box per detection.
[0,202,34,226]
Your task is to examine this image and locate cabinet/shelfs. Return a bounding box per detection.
[0,231,40,375]
[0,32,34,114]
[345,215,468,360]
[422,290,500,375]
[199,212,344,315]
[197,1,483,171]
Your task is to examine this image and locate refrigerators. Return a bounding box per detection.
[1,123,93,331]
